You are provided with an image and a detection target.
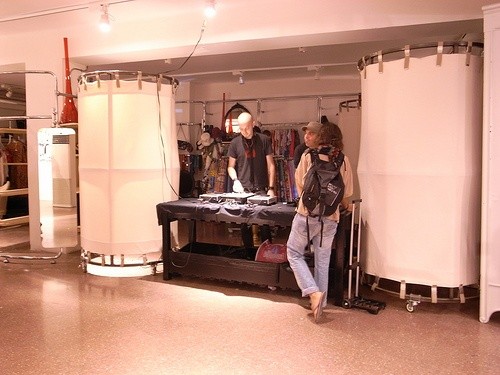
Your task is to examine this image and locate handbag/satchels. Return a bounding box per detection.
[177,125,193,154]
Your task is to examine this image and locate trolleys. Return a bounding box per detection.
[342,198,387,316]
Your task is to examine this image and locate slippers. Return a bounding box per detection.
[311,291,326,323]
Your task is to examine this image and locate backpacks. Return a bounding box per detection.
[303,147,344,217]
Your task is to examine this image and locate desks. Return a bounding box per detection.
[156,199,352,306]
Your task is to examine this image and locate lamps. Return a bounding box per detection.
[239,75,244,84]
[204,0,216,17]
[314,66,322,80]
[99,4,112,31]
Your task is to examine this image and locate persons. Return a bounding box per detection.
[227,112,277,247]
[287,122,354,322]
[293,121,323,168]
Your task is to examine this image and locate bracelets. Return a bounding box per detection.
[268,187,274,190]
[233,178,238,181]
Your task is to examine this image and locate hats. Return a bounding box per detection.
[301,122,323,133]
[196,126,224,150]
